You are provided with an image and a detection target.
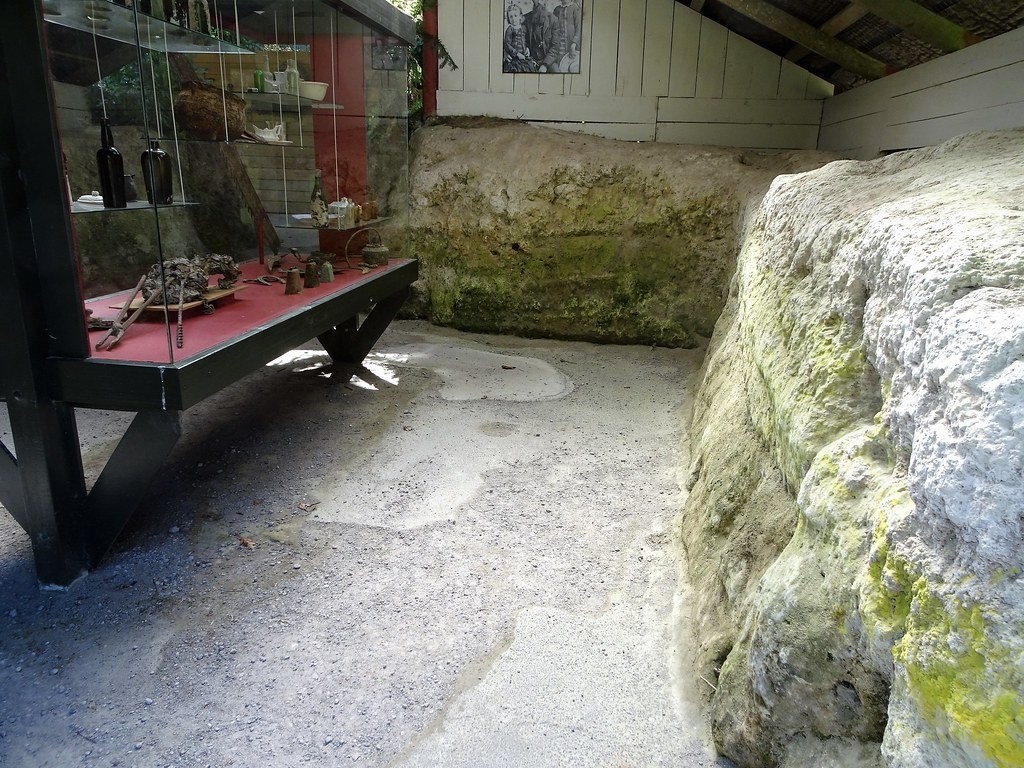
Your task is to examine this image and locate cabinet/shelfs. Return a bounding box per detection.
[2,0,422,585]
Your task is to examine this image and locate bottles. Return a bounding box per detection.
[285,57,298,93]
[142,139,173,204]
[254,65,265,91]
[263,47,273,91]
[346,198,355,226]
[97,115,127,208]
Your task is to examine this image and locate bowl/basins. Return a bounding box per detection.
[298,79,329,101]
